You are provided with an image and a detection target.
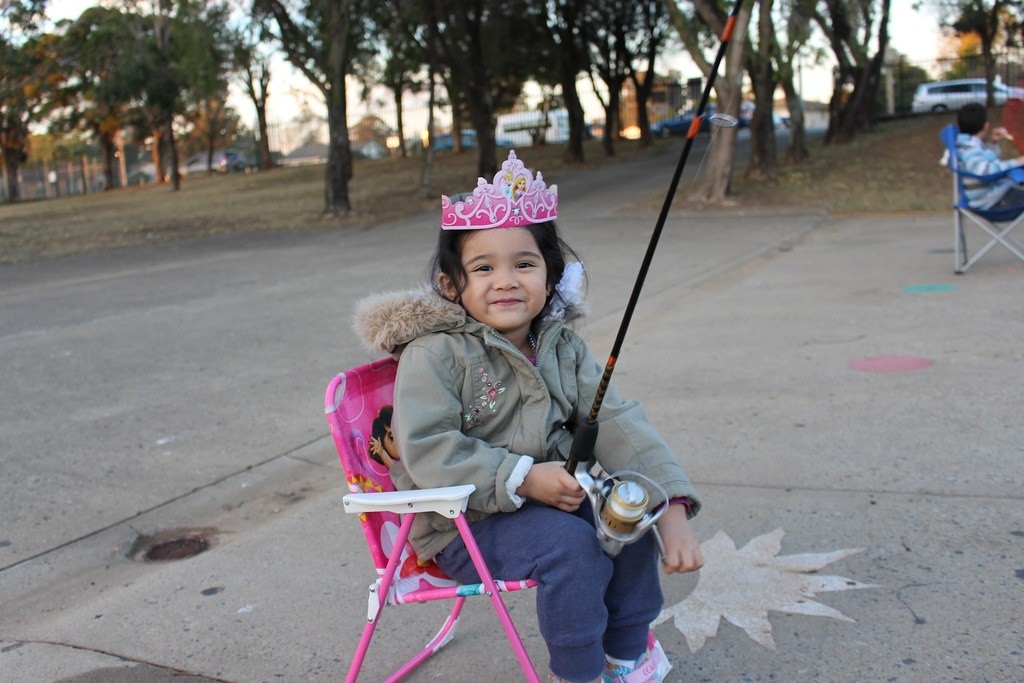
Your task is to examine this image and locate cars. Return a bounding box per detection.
[650,98,784,137]
[913,76,1024,112]
[433,129,515,150]
[31,147,245,199]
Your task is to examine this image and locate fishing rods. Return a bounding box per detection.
[565,0,746,561]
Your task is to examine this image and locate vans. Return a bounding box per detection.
[496,106,569,147]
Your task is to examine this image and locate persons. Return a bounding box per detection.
[955,101,1024,223]
[388,196,704,683]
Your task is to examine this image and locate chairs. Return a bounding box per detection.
[324,356,541,683]
[939,122,1024,274]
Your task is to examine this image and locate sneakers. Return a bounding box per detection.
[553,645,661,683]
